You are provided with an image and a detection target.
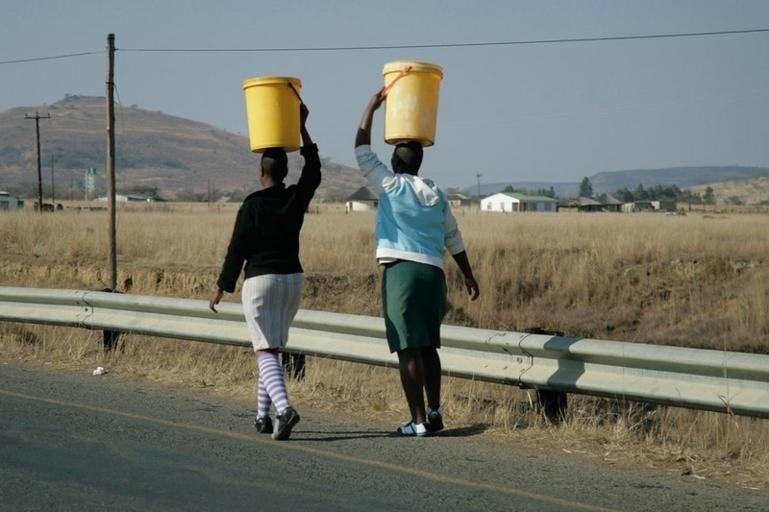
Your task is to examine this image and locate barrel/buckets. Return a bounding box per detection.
[382,62,444,148]
[241,75,305,154]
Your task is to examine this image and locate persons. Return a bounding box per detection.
[352,90,481,439]
[207,100,324,442]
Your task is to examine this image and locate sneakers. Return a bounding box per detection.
[271,406,301,441]
[396,419,430,436]
[427,404,446,433]
[253,414,273,433]
